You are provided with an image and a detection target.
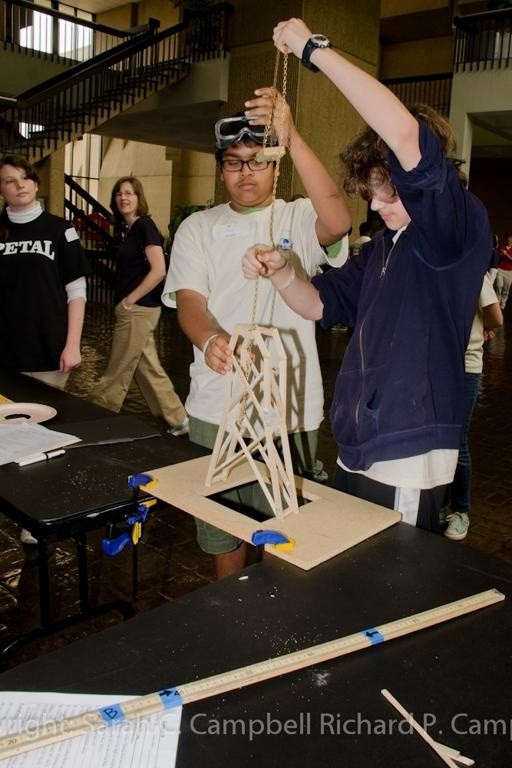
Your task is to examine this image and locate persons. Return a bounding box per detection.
[240,17,491,538]
[158,86,354,580]
[0,154,90,392]
[83,176,188,437]
[321,222,511,541]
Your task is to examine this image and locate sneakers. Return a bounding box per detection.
[439,509,471,540]
[165,418,191,436]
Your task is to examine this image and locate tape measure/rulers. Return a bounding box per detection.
[0,588,505,762]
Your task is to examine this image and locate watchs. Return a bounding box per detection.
[300,33,332,72]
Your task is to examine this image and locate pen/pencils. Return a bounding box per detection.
[18,450,67,467]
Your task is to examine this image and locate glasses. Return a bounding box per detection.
[214,115,279,146]
[220,156,270,172]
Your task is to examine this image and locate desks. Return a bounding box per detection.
[0,368,512,768]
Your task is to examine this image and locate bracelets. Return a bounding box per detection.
[202,333,219,352]
[123,298,132,310]
[279,262,295,291]
